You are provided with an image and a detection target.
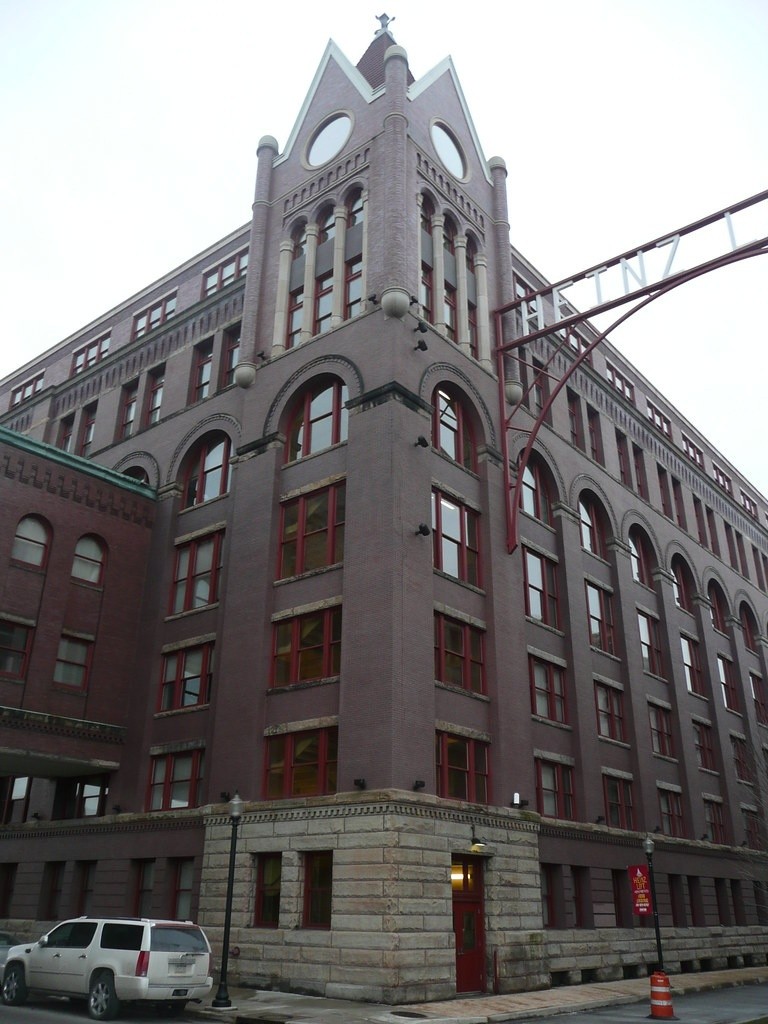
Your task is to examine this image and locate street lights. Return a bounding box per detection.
[643,834,666,972]
[204,794,243,1012]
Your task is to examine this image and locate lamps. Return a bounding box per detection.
[415,523,431,536]
[512,792,529,809]
[413,320,429,332]
[653,825,661,833]
[414,435,430,448]
[467,824,487,853]
[112,805,121,816]
[412,780,425,792]
[354,777,365,788]
[32,812,42,818]
[219,791,230,801]
[741,840,747,847]
[595,814,606,825]
[415,341,430,352]
[701,833,708,840]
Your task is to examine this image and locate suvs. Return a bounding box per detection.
[1,915,214,1021]
